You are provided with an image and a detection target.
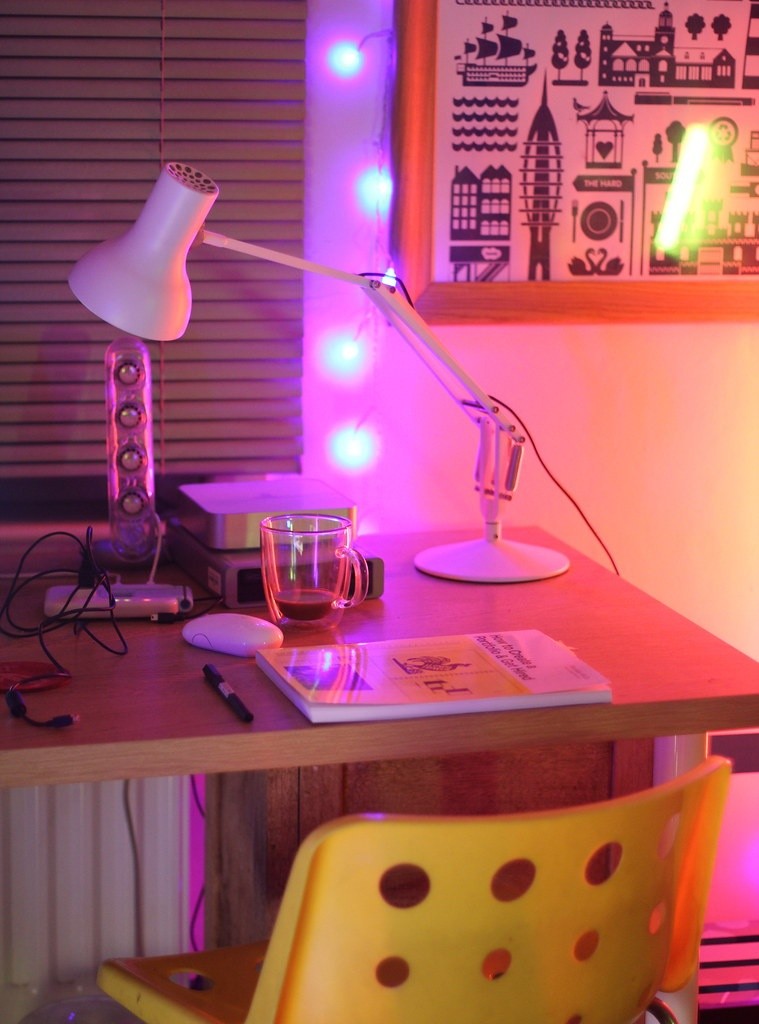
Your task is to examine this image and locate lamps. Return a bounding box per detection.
[69,162,573,582]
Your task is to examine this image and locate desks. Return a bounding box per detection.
[0,524,758,1024]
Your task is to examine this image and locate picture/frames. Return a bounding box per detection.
[388,0,759,328]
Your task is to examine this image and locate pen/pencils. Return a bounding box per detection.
[203,662,254,722]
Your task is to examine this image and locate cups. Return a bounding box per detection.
[260,513,369,636]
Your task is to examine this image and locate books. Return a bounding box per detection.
[256,629,611,722]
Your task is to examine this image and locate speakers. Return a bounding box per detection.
[103,335,158,564]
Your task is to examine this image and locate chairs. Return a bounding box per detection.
[96,754,733,1024]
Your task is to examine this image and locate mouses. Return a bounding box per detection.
[182,613,283,657]
